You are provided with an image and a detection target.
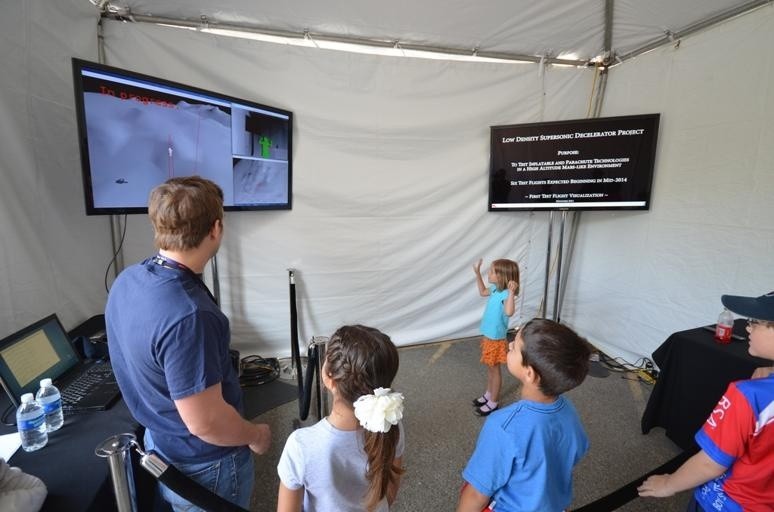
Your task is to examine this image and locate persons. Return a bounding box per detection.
[453,316,592,511]
[470,256,521,416]
[272,323,406,511]
[636,291,772,511]
[104,175,274,511]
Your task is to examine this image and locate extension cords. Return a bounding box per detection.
[638,369,657,386]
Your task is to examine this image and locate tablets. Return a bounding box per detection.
[0,312,123,415]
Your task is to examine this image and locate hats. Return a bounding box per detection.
[722,291,774,321]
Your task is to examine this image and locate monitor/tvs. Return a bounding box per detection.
[489,115,661,211]
[72,57,292,215]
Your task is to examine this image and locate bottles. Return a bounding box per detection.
[11,391,46,451]
[36,377,63,432]
[714,309,735,345]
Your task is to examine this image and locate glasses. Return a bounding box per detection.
[747,317,774,328]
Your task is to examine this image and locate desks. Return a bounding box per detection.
[639,317,774,462]
[0,312,145,512]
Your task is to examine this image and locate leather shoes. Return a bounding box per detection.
[471,395,488,406]
[473,403,498,416]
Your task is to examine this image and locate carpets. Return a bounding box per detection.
[241,377,300,422]
